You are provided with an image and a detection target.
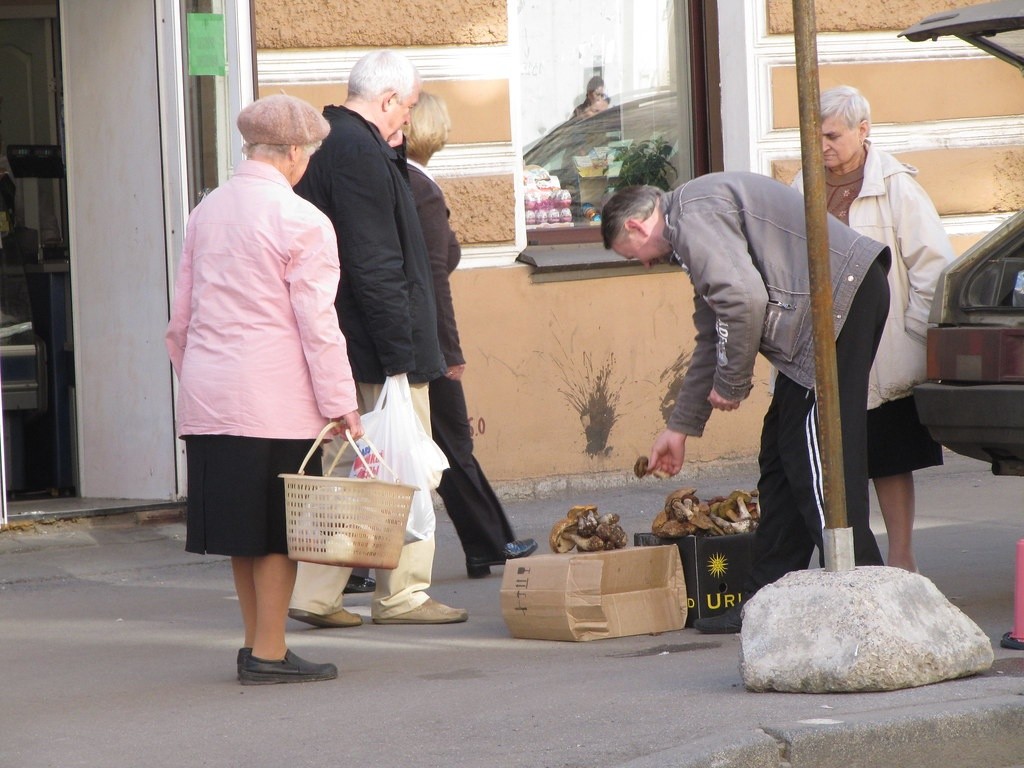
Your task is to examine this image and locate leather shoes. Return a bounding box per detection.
[241,649,336,685]
[342,574,377,594]
[236,646,253,678]
[467,538,537,579]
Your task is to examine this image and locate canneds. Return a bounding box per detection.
[582,203,601,221]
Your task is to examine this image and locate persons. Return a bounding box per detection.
[601,172,892,635]
[288,50,468,628]
[165,94,365,686]
[342,92,538,593]
[561,77,619,222]
[767,85,958,574]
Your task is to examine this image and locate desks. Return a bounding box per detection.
[1,259,73,499]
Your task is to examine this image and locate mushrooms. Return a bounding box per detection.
[549,504,628,553]
[650,487,761,539]
[633,456,670,480]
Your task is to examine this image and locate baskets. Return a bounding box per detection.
[277,421,422,569]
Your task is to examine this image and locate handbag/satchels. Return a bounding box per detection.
[348,373,450,545]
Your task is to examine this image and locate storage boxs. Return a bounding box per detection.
[635,530,756,627]
[501,544,689,641]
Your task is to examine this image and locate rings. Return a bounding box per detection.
[450,372,453,375]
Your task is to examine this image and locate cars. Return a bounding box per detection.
[897,2,1024,478]
[521,82,692,223]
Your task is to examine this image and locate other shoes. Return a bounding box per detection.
[693,593,753,633]
[373,597,467,623]
[288,607,364,627]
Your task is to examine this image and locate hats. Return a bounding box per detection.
[237,88,331,145]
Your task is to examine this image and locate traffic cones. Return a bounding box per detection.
[999,538,1024,650]
